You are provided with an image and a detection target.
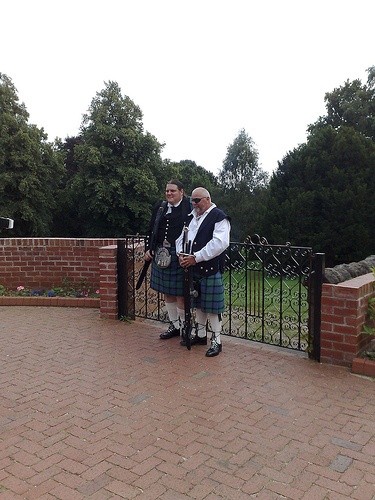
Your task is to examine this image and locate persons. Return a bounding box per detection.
[145,179,193,339]
[176,187,232,357]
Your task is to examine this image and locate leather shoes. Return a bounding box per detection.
[180,334,208,346]
[180,328,193,340]
[206,341,222,356]
[160,324,181,340]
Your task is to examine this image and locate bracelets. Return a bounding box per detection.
[190,255,198,263]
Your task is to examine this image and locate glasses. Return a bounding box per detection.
[190,197,206,203]
